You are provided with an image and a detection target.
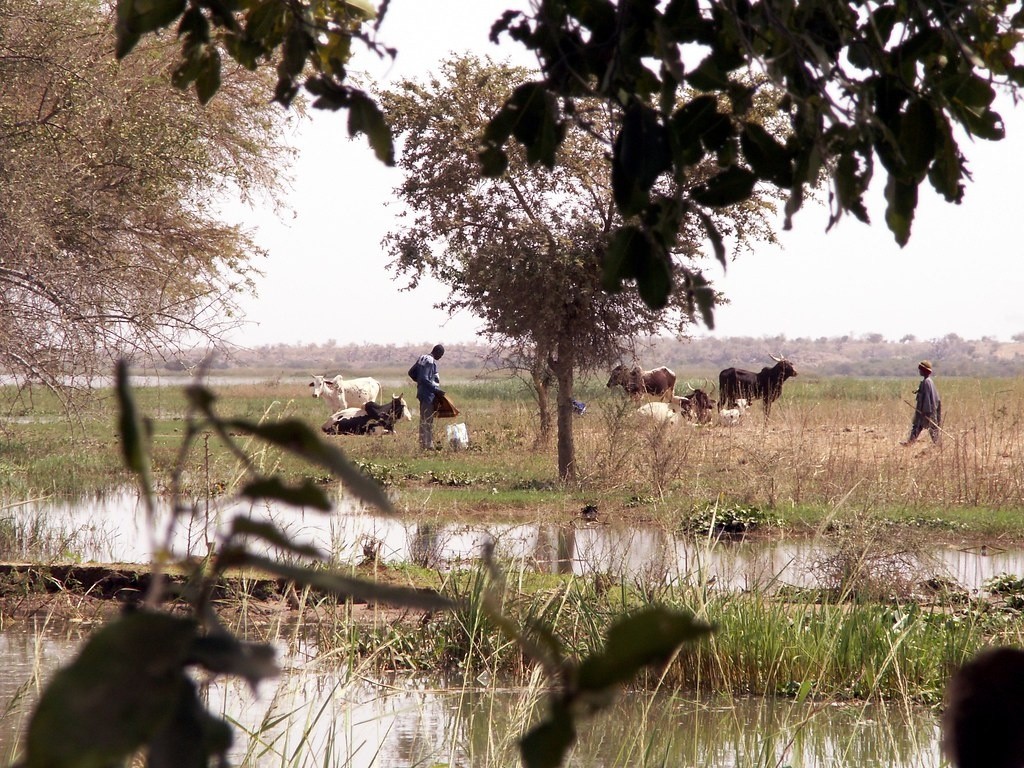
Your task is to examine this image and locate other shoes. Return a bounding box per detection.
[900,441,913,447]
[420,446,440,456]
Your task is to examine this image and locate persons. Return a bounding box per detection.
[898,360,940,447]
[408,344,445,452]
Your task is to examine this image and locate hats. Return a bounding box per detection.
[917,360,933,372]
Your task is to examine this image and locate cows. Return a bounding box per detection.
[635,386,691,429]
[605,364,677,404]
[309,373,382,417]
[321,393,412,436]
[717,350,799,422]
[717,398,753,425]
[677,377,716,426]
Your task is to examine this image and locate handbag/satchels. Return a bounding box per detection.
[448,423,469,453]
[433,393,459,417]
